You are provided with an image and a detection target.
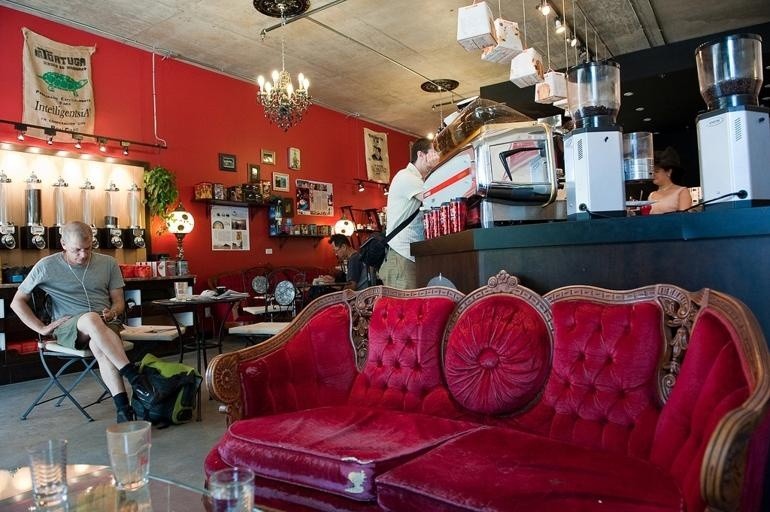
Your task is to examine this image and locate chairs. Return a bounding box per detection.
[21,291,114,421]
[208,264,307,354]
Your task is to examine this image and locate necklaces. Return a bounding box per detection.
[658,181,676,193]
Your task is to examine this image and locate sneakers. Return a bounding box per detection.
[131,376,159,403]
[117,409,137,422]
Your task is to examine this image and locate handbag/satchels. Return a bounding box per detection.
[131,352,204,429]
[360,231,389,269]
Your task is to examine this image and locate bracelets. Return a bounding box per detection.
[105,311,118,325]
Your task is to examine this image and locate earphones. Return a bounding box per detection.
[62,242,66,247]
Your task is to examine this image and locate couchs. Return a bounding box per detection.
[204,268,770,511]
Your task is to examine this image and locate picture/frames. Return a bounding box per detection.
[260,148,275,165]
[288,147,301,171]
[282,197,294,217]
[247,163,260,185]
[219,152,236,171]
[273,172,289,192]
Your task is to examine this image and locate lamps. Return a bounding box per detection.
[536,0,587,58]
[358,182,365,192]
[383,187,389,196]
[457,1,571,117]
[14,123,131,156]
[165,201,194,261]
[253,0,313,132]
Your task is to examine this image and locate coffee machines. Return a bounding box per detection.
[693,33,769,209]
[561,62,629,222]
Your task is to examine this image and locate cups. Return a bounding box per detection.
[104,418,154,489]
[207,468,256,512]
[23,438,69,499]
[174,280,190,300]
[31,498,69,511]
[115,485,155,512]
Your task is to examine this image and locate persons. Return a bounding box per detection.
[377,135,440,291]
[8,220,160,425]
[647,148,693,215]
[307,233,369,302]
